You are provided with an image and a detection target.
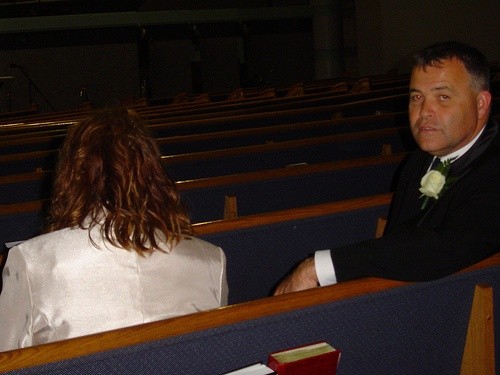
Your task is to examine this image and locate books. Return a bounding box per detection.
[219,361,277,375]
[267,340,342,375]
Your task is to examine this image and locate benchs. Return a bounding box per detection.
[0,73,416,306]
[0,252,500,375]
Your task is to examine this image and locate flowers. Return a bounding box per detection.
[419,157,459,209]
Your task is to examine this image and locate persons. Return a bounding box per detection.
[273,36,500,296]
[0,105,230,354]
